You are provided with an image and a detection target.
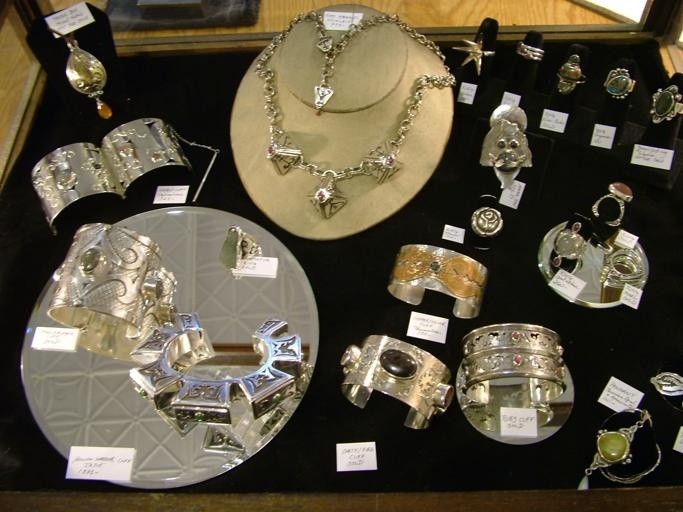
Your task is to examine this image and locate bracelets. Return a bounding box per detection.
[32,118,220,227]
[46,223,177,336]
[386,244,489,318]
[128,313,309,423]
[461,323,568,408]
[340,334,454,429]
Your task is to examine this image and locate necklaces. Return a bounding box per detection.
[40,13,115,121]
[256,9,457,219]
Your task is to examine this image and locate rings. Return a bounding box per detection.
[557,45,588,93]
[649,72,683,125]
[478,104,533,189]
[603,58,637,98]
[600,248,646,288]
[592,182,633,227]
[577,408,662,490]
[551,222,587,266]
[516,29,543,61]
[471,194,503,251]
[451,18,499,76]
